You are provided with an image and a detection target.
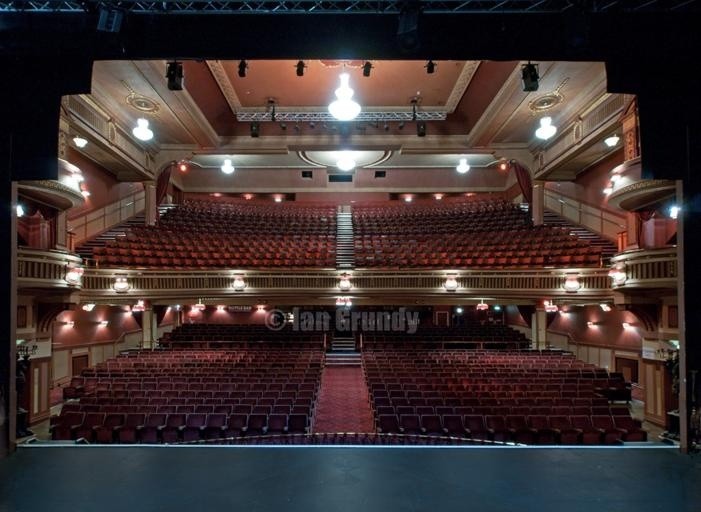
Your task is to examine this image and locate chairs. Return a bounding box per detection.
[51,321,647,449]
[89,196,609,270]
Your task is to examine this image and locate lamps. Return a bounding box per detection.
[130,98,160,143]
[73,132,86,150]
[162,60,548,92]
[532,95,560,141]
[604,129,621,150]
[324,68,365,125]
